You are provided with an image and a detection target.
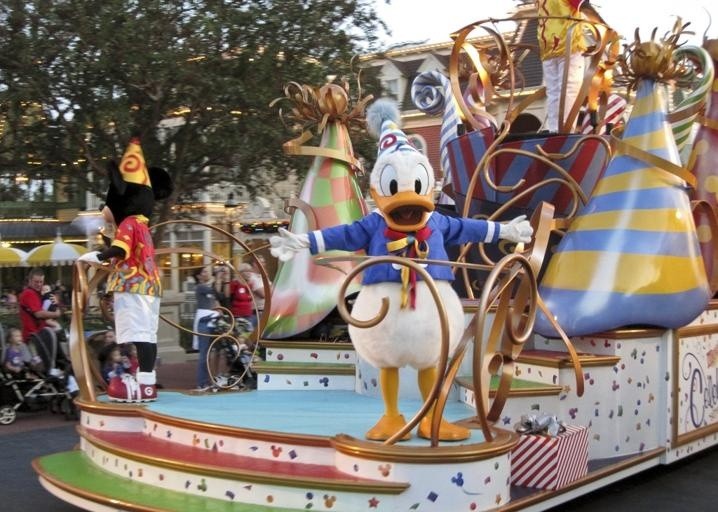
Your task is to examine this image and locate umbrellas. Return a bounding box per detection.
[20,236,91,280]
[0,247,27,268]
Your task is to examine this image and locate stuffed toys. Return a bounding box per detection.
[269,99,534,441]
[77,126,174,403]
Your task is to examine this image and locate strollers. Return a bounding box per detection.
[208,314,257,390]
[0,324,163,425]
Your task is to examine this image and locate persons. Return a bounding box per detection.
[0,267,138,411]
[192,254,272,392]
[537,0,585,135]
